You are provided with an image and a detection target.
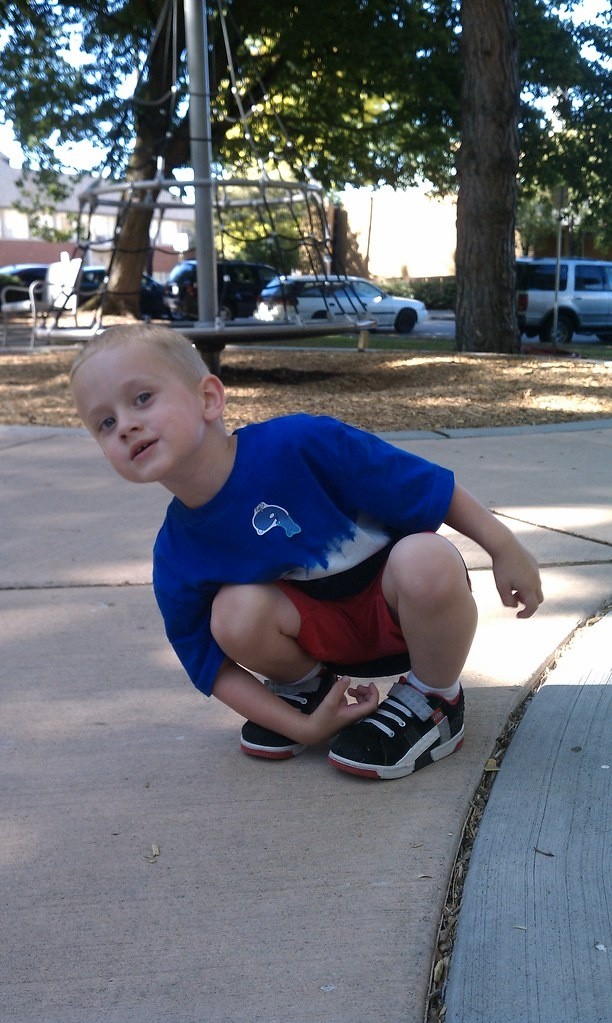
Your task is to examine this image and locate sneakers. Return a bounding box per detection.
[328,677,465,780]
[240,664,338,760]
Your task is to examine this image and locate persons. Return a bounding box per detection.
[67,321,546,785]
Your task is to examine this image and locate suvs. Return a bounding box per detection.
[514,255,612,345]
[162,258,286,326]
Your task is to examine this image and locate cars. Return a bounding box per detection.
[252,274,429,334]
[77,266,164,320]
[0,263,51,307]
